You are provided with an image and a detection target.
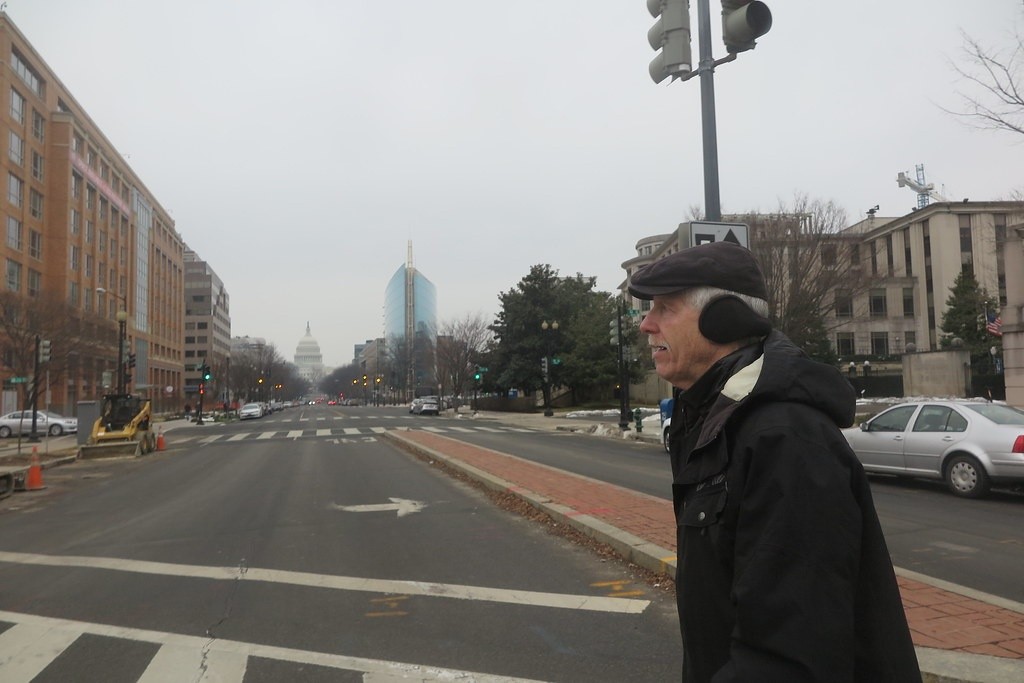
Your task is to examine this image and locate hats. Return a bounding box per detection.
[625,241,766,298]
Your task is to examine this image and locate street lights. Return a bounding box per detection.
[541,319,559,377]
[226,353,231,404]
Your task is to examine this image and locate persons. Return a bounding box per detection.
[185,402,192,421]
[628,242,925,683]
[195,401,200,419]
[223,402,227,414]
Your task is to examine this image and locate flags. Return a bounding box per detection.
[987,305,1002,336]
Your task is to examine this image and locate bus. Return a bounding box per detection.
[0,471,16,500]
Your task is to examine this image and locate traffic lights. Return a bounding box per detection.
[647,0,773,85]
[609,315,628,345]
[475,373,480,382]
[542,357,548,373]
[116,310,136,385]
[38,339,52,364]
[199,365,210,394]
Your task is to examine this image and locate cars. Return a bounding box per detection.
[836,401,1024,497]
[409,399,439,415]
[0,409,78,438]
[310,400,315,404]
[240,401,299,421]
[659,418,671,453]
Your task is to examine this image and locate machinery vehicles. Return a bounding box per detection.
[75,393,155,462]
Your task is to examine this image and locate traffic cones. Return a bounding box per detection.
[25,446,48,490]
[155,426,167,452]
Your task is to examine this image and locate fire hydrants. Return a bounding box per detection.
[634,407,644,432]
